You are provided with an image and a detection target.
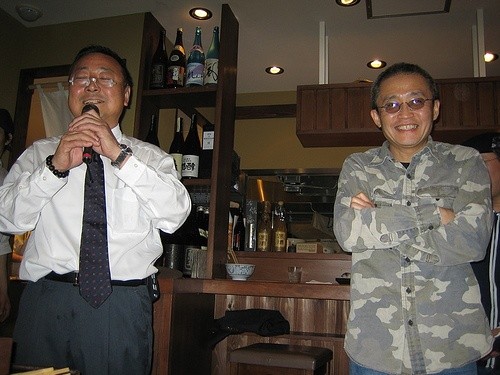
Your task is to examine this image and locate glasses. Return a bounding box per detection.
[377,98,434,114]
[68,72,127,89]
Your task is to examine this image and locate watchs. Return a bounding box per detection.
[111,144,133,167]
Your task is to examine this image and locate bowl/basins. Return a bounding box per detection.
[225,264,256,281]
[336,277,351,285]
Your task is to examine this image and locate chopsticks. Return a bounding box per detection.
[230,249,239,264]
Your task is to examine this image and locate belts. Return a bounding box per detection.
[45,271,147,287]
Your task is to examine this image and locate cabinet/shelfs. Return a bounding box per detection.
[132,3,240,279]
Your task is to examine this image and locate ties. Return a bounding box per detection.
[77,152,113,307]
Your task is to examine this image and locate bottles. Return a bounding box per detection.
[149,30,168,89]
[186,26,205,88]
[169,110,184,177]
[288,209,295,238]
[257,201,272,252]
[272,200,287,252]
[167,27,187,88]
[181,111,201,179]
[245,199,257,252]
[233,208,246,252]
[144,112,160,148]
[228,211,232,250]
[160,202,210,248]
[203,26,220,88]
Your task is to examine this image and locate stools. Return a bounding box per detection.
[229,343,333,375]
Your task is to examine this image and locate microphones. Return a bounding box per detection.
[80,104,100,164]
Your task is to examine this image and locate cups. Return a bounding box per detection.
[287,264,302,284]
[184,247,208,279]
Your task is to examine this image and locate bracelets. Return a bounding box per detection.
[46,155,70,178]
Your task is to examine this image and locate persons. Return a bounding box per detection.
[0,46,191,375]
[0,107,14,321]
[463,131,500,375]
[333,62,495,375]
